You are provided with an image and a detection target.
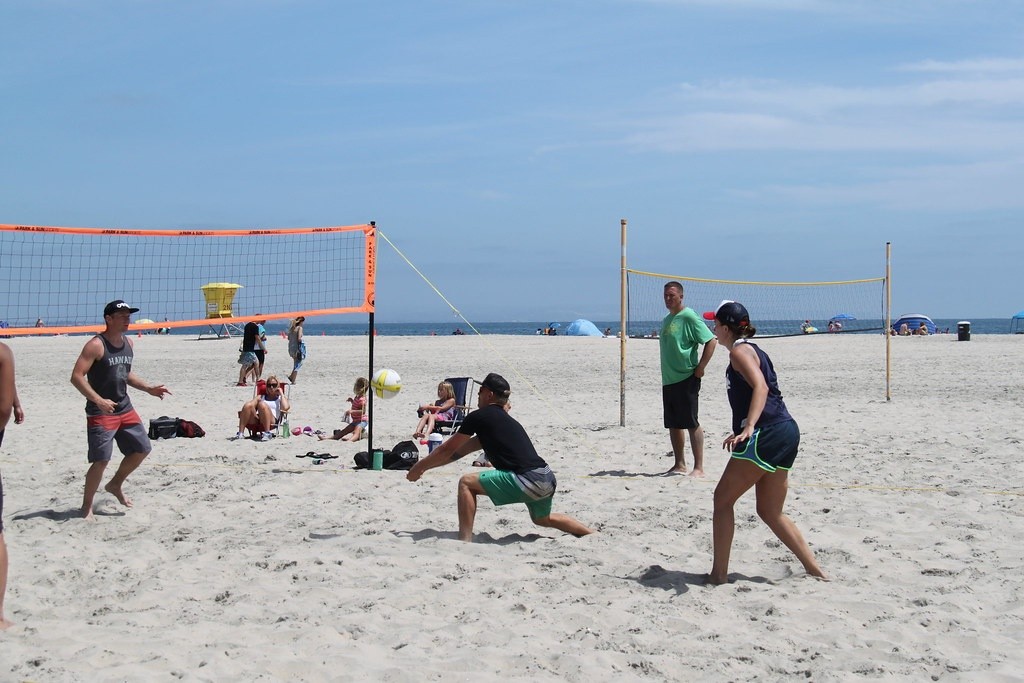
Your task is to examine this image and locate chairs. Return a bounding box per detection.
[418,377,474,438]
[239,382,290,438]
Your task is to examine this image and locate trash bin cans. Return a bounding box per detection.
[957,321,971,341]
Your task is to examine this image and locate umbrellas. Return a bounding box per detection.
[832,313,857,327]
[135,318,154,323]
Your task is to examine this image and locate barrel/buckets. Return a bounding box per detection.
[428,432,443,453]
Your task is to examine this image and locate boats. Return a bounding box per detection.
[830,313,857,321]
[893,313,936,334]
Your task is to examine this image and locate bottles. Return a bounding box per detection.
[282,422,290,439]
[373,447,383,471]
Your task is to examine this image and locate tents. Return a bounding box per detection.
[1009,310,1024,334]
[894,313,936,334]
[565,319,603,336]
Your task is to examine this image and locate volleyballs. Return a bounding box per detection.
[371,368,403,400]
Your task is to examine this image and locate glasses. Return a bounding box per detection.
[266,383,278,388]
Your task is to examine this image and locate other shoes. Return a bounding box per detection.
[262,433,271,441]
[236,435,245,440]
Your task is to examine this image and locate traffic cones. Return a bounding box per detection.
[279,331,287,339]
[321,331,324,336]
[373,328,377,336]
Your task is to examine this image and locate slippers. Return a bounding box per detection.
[298,452,338,459]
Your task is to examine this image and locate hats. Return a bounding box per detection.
[473,373,511,399]
[702,300,749,326]
[104,300,139,319]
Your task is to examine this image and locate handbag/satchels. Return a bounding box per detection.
[381,441,418,471]
[175,419,206,438]
[148,416,182,439]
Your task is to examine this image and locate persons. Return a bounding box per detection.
[0,342,24,634]
[317,377,368,442]
[802,320,811,334]
[157,317,171,335]
[704,300,824,579]
[834,320,841,334]
[899,321,912,335]
[69,300,173,522]
[660,281,717,479]
[34,318,45,337]
[235,376,290,441]
[604,328,611,335]
[916,322,928,335]
[828,321,834,333]
[236,313,305,385]
[406,373,592,540]
[535,322,556,335]
